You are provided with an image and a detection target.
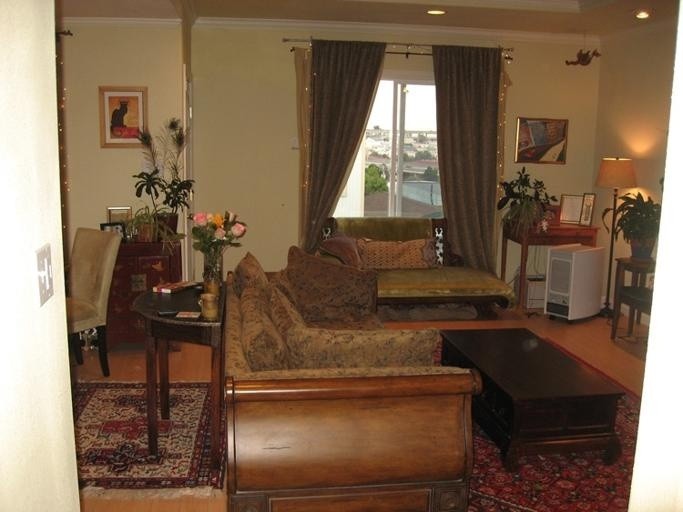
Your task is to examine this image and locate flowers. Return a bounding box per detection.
[186,208,247,276]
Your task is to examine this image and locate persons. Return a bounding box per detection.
[115,227,123,239]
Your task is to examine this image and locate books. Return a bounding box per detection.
[152,280,197,294]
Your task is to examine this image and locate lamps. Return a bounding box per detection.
[596,157,638,318]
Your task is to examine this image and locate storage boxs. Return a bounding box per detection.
[515,275,546,309]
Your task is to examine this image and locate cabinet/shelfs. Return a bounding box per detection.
[108,242,182,344]
[610,258,655,340]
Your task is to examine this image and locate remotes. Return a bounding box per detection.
[195,282,222,291]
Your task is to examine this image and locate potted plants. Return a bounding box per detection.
[100,118,195,257]
[602,191,661,268]
[496,166,556,237]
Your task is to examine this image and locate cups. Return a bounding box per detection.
[199,293,217,318]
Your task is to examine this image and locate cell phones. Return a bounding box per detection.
[158,309,178,316]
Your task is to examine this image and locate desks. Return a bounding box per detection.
[131,282,225,470]
[501,224,598,320]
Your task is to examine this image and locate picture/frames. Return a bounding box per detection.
[99,85,148,148]
[580,193,596,227]
[514,116,569,166]
[557,194,585,225]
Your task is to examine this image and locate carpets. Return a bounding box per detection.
[71,381,227,500]
[465,336,641,511]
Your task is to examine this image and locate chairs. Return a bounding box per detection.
[65,228,123,377]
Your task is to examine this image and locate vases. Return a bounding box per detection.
[203,276,220,302]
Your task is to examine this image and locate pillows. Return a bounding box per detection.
[235,245,444,372]
[321,232,436,269]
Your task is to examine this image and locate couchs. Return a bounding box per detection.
[317,217,513,320]
[225,270,482,511]
